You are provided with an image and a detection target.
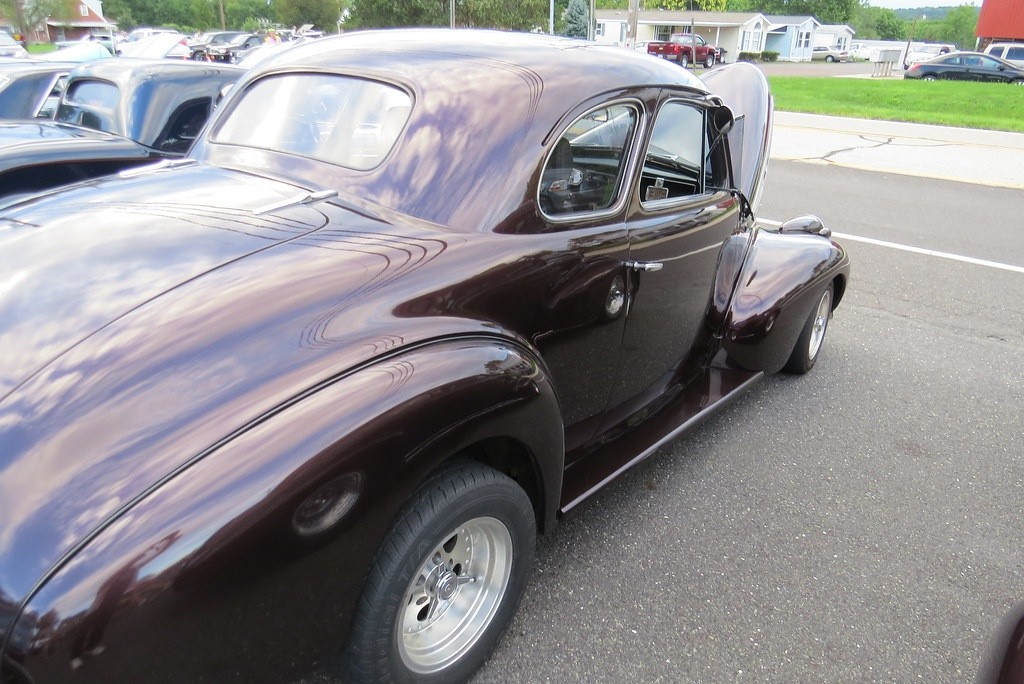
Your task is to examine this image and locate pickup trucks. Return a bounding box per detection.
[646,32,718,70]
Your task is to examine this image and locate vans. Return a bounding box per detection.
[977,43,1024,72]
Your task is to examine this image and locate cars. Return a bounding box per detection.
[0,28,325,218]
[849,42,892,63]
[713,46,728,63]
[634,40,666,59]
[904,44,957,70]
[904,51,1024,87]
[811,44,849,63]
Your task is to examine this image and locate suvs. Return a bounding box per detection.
[0,26,849,684]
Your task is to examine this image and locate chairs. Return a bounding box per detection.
[540,137,606,216]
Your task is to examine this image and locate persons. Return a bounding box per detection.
[116,32,129,40]
[265,29,281,45]
[87,30,95,40]
